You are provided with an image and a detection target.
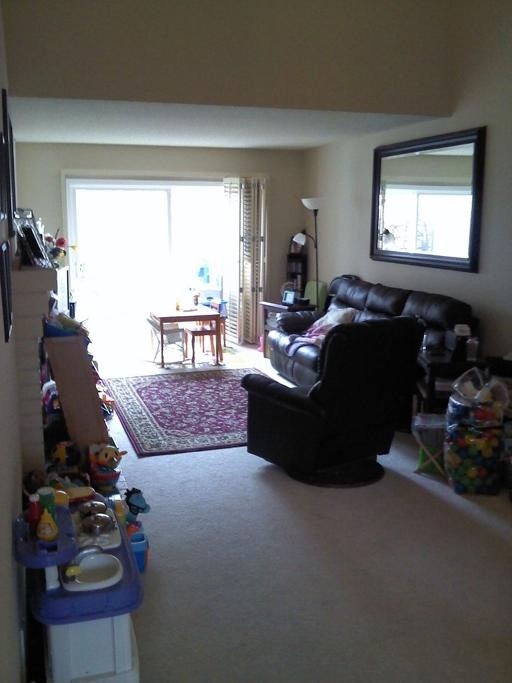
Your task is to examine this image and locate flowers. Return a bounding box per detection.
[44,227,77,259]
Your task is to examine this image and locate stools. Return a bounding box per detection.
[412,412,448,479]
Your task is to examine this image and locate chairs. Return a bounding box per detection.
[239,316,426,488]
[142,301,223,365]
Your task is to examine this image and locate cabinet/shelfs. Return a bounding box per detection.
[42,332,107,452]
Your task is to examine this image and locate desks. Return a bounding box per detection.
[258,301,318,358]
[198,297,227,346]
[411,357,512,435]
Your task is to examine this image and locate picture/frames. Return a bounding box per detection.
[0,243,14,343]
[14,216,53,269]
[2,85,16,236]
[289,236,304,254]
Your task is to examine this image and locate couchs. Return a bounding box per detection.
[265,275,473,432]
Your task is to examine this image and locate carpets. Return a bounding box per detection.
[101,368,287,456]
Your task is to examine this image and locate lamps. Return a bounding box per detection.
[292,196,326,310]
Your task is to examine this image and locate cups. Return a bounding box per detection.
[465,335,480,362]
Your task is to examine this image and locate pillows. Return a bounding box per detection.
[300,303,356,336]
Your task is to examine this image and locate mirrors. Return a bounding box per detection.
[370,125,488,271]
